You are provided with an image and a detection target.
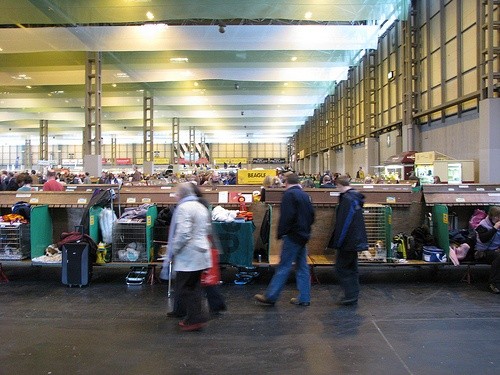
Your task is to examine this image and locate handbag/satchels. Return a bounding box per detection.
[200,250,221,287]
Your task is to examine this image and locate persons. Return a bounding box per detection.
[323,178,368,304]
[433,176,443,184]
[254,173,315,306]
[159,182,225,331]
[269,165,364,188]
[0,165,237,191]
[474,207,500,294]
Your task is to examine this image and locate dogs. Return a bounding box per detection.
[362,240,387,262]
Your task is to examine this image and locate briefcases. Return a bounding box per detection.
[60,224,92,289]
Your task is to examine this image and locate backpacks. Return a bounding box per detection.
[470,209,488,229]
[412,225,434,259]
[13,200,32,221]
[394,232,415,259]
[421,245,448,263]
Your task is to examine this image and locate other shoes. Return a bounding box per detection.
[165,309,185,319]
[255,293,276,304]
[488,283,500,292]
[179,320,209,330]
[290,298,311,305]
[208,305,228,312]
[340,296,359,304]
